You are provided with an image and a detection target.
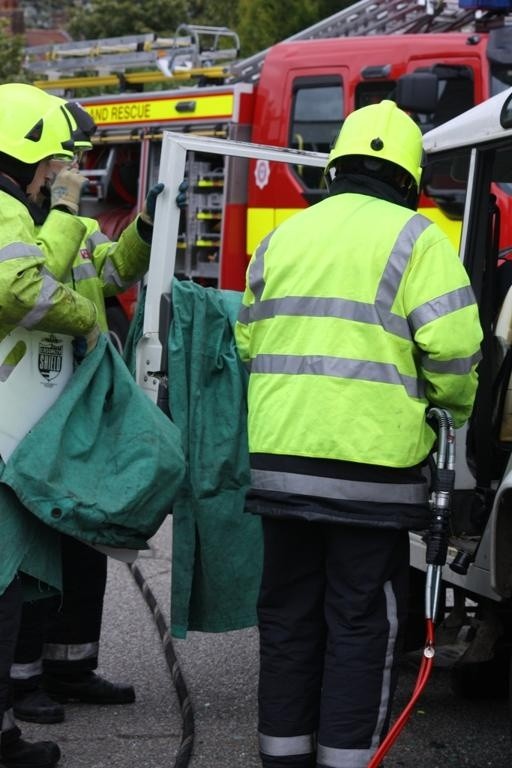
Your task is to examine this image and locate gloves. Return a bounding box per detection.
[50,167,89,215]
[71,325,101,365]
[139,181,189,226]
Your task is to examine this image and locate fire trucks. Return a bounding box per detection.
[13,0,510,360]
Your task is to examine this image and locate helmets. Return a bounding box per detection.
[323,99,423,195]
[0,83,98,164]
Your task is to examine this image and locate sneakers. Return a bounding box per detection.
[12,671,136,724]
[0,725,60,768]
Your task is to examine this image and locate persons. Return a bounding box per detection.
[234,103,485,768]
[1,80,186,767]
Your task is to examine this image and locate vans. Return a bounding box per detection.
[119,88,512,708]
